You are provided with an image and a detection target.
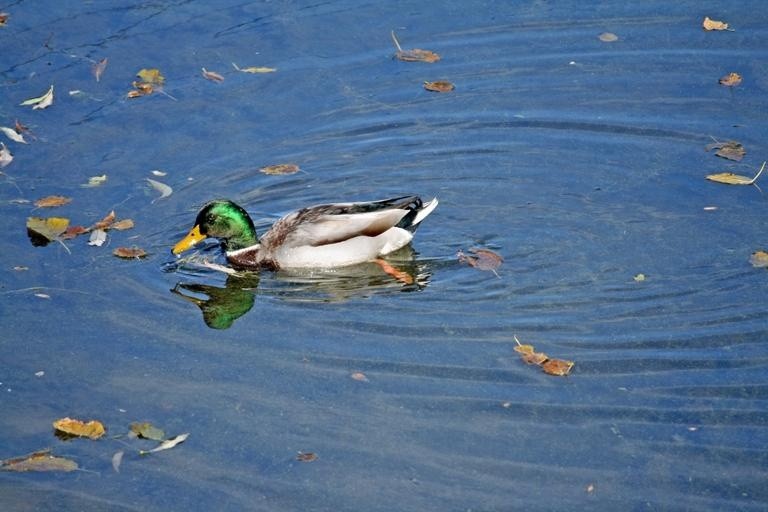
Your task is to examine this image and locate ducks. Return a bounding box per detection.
[170,194,437,284]
